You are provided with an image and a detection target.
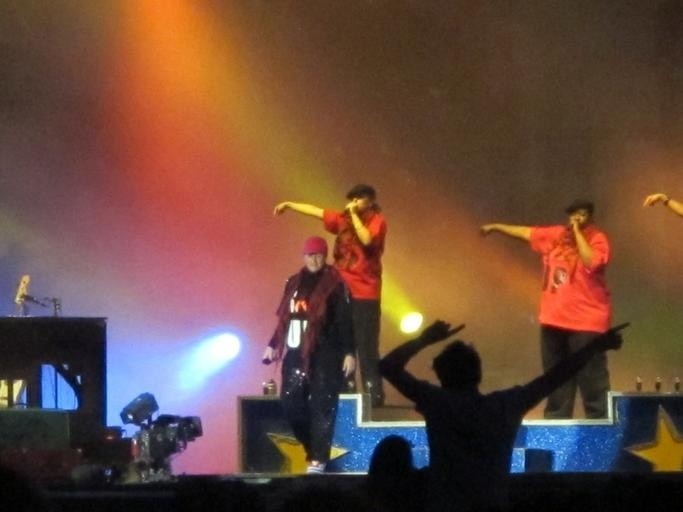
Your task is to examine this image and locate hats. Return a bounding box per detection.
[565,199,594,216]
[304,236,328,257]
[347,184,376,201]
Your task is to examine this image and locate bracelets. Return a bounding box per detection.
[663,195,671,208]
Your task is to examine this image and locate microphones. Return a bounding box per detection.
[340,208,349,217]
[567,217,579,231]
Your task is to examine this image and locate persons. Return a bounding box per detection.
[376,316,632,475]
[642,192,683,216]
[479,198,612,420]
[263,234,358,473]
[271,182,386,408]
